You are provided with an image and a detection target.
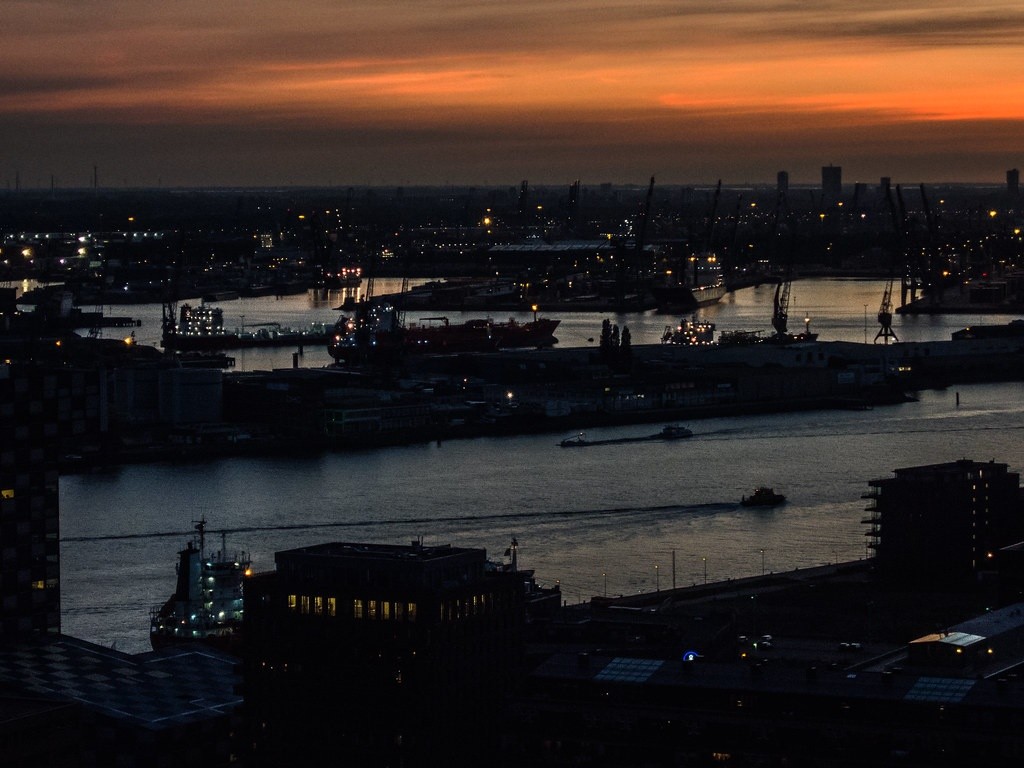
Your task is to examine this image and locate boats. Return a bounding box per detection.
[660,424,692,439]
[740,488,787,510]
[148,518,271,650]
[561,433,590,447]
[160,240,827,356]
